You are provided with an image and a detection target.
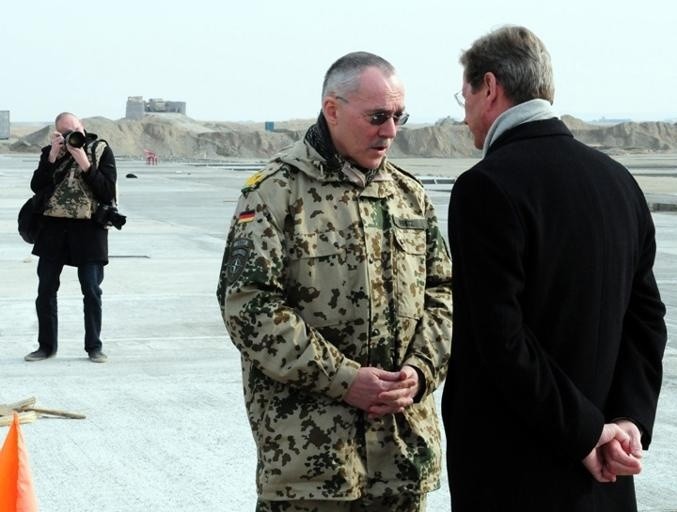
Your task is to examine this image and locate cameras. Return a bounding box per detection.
[60,130,86,149]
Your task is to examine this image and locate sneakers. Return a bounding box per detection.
[25,347,57,362]
[89,347,108,362]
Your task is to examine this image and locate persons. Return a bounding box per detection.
[23,110,126,363]
[215,49,454,511]
[440,24,669,512]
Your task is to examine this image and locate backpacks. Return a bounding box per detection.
[17,192,45,245]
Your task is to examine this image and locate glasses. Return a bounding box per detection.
[337,96,410,126]
[454,89,465,106]
